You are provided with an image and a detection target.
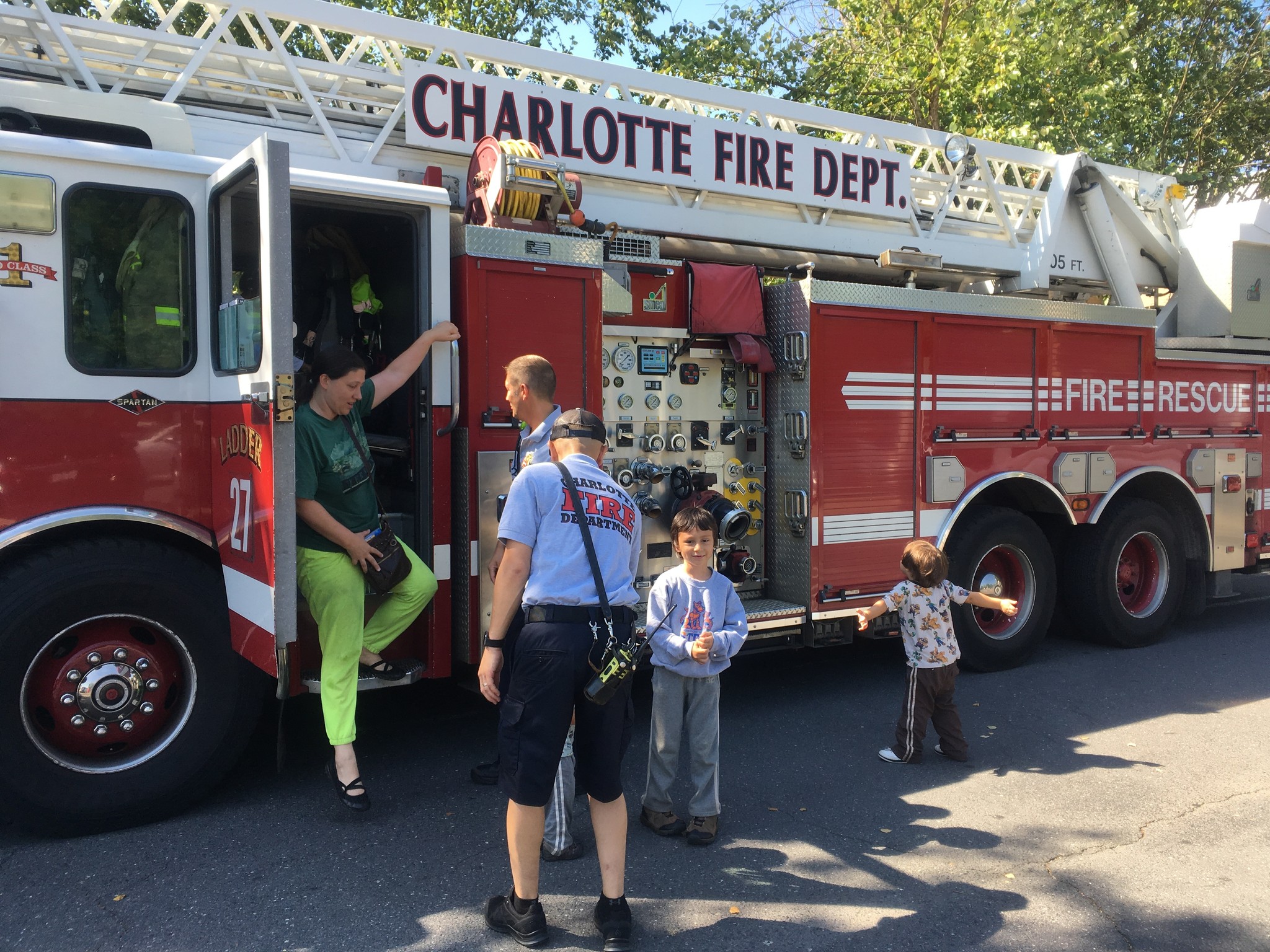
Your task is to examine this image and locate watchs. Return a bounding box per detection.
[483,630,505,649]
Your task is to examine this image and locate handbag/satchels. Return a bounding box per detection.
[344,521,412,597]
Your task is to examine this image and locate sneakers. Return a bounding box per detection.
[484,884,552,947]
[685,815,719,844]
[934,744,948,757]
[878,748,907,763]
[640,805,687,835]
[593,899,633,951]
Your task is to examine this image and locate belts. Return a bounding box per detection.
[525,604,638,625]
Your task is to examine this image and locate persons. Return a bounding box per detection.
[471,352,564,784]
[645,506,749,842]
[476,408,648,952]
[539,704,582,860]
[295,320,462,808]
[856,540,1018,764]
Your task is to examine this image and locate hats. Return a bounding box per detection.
[549,408,606,457]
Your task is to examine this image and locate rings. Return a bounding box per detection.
[483,683,488,687]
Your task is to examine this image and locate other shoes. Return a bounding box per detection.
[324,760,371,812]
[540,842,584,862]
[359,659,406,681]
